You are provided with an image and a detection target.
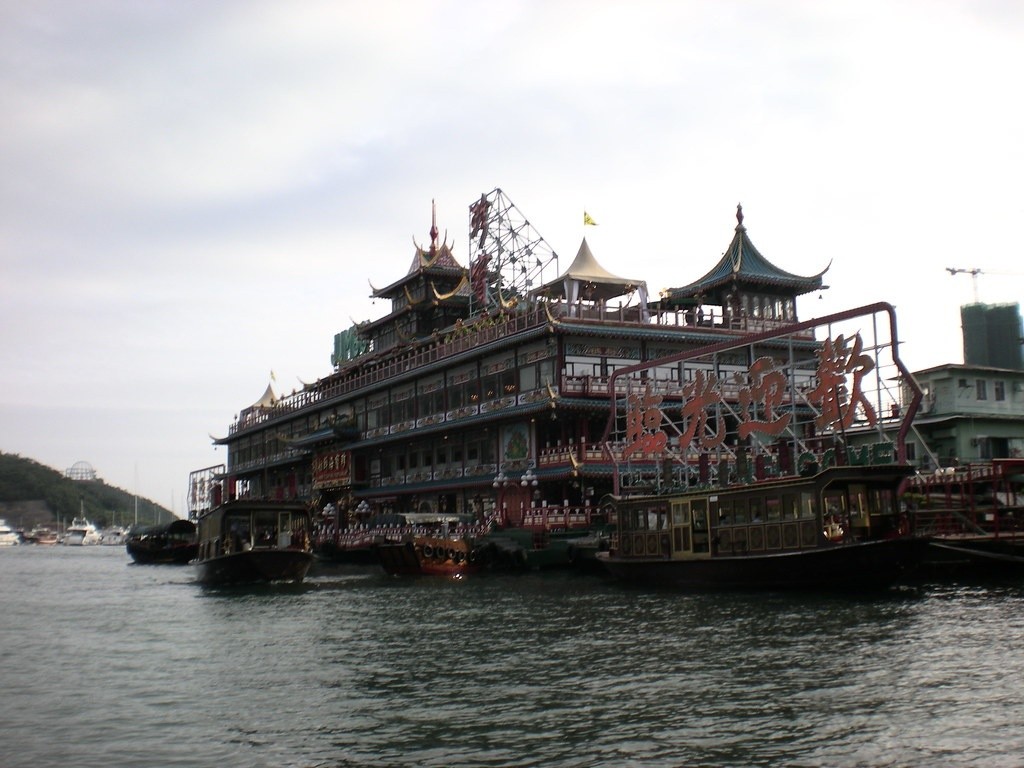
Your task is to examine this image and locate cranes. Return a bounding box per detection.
[945,266,987,303]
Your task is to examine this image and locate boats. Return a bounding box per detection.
[594,389,1024,579]
[0,491,131,546]
[126,498,558,583]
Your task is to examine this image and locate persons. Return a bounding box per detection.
[697,306,704,321]
[685,308,693,323]
[752,512,762,522]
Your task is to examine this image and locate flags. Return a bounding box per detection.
[584,212,599,225]
